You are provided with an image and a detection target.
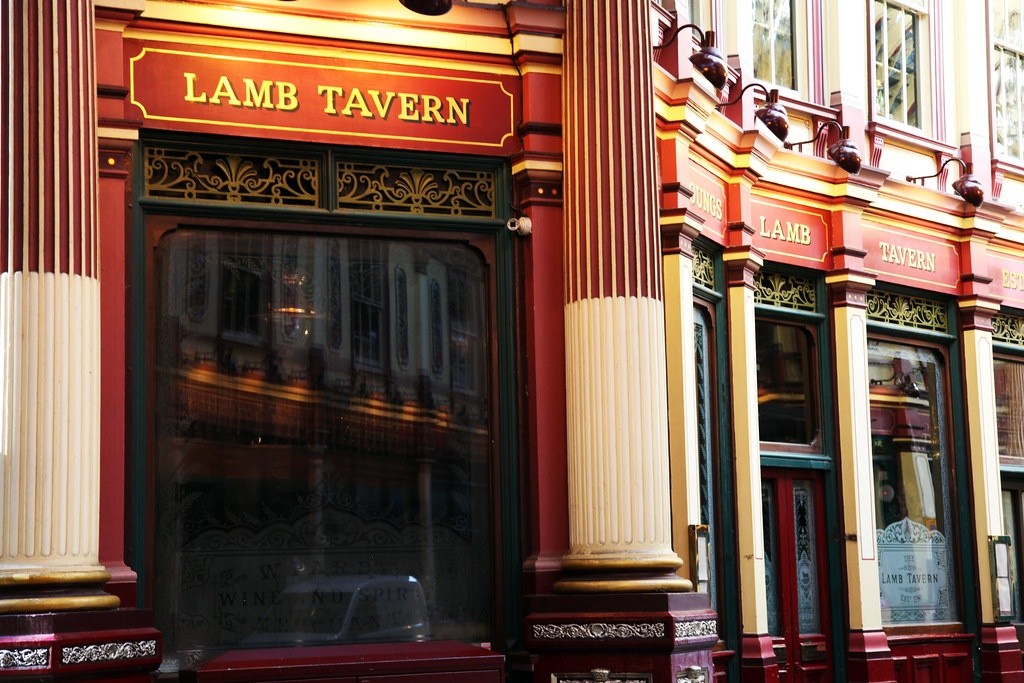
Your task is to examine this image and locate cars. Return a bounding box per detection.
[230,574,435,649]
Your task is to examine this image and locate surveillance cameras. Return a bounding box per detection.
[506,216,532,236]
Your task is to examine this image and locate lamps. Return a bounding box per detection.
[905,158,983,208]
[653,23,725,90]
[713,83,790,143]
[782,121,862,176]
